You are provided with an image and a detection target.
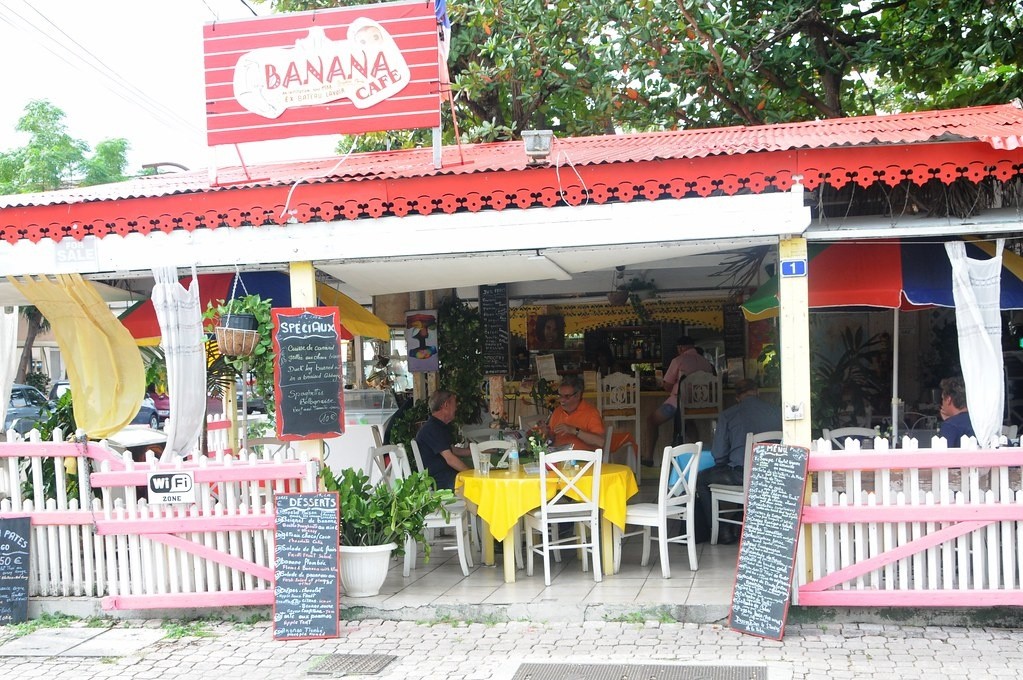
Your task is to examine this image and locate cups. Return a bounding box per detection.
[478,454,490,475]
[562,448,571,470]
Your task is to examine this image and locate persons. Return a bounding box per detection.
[697,378,782,543]
[539,377,606,535]
[536,315,566,347]
[592,346,623,375]
[937,374,976,448]
[415,389,498,517]
[641,336,713,466]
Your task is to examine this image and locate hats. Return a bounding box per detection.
[673,336,694,346]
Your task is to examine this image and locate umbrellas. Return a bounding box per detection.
[115,271,390,448]
[738,234,1023,449]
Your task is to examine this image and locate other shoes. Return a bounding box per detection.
[522,541,526,548]
[733,534,742,538]
[558,524,570,534]
[494,544,503,554]
[640,455,654,467]
[717,538,733,544]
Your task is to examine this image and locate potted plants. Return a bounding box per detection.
[197,285,277,458]
[617,276,658,325]
[317,460,453,597]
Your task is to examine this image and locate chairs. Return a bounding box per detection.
[525,446,603,586]
[409,439,480,561]
[882,411,938,447]
[612,439,702,579]
[823,425,884,459]
[371,444,474,578]
[569,424,614,537]
[709,431,785,546]
[468,437,535,573]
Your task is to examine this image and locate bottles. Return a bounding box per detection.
[609,340,660,360]
[509,439,519,472]
[497,431,505,456]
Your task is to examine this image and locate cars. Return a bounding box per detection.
[212,372,277,414]
[48,379,159,431]
[3,383,58,436]
[147,385,224,428]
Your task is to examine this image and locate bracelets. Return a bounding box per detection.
[575,428,579,436]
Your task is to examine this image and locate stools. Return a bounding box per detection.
[596,368,643,485]
[677,371,723,458]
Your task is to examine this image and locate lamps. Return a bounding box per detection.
[519,128,552,163]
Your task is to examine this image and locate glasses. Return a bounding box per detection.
[557,392,577,400]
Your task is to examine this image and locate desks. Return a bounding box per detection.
[451,463,640,584]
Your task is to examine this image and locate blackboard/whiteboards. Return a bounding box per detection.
[728,441,809,641]
[479,283,511,377]
[271,492,339,642]
[273,306,345,440]
[0,516,30,626]
